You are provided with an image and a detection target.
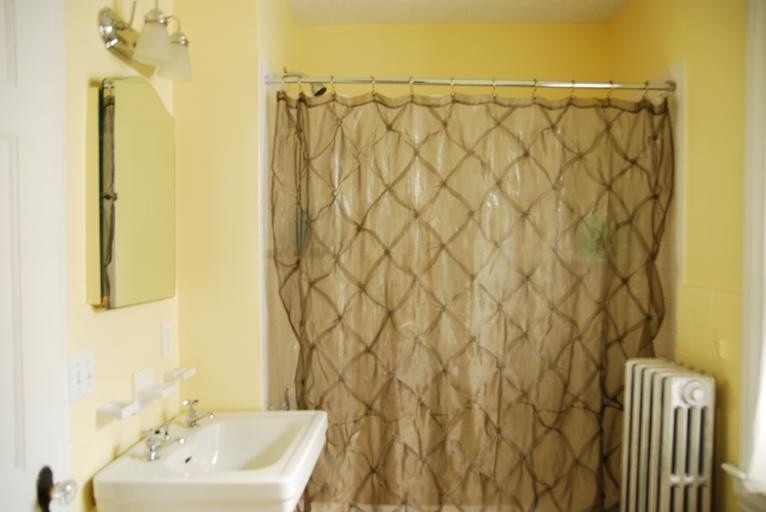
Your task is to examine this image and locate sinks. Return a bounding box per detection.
[91,410,327,511]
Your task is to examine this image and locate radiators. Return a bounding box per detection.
[618,357,716,512]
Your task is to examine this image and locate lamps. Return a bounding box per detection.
[98,0,194,80]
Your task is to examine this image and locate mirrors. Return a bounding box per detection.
[90,78,182,312]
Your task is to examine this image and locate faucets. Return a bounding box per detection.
[148,427,184,457]
[176,396,215,427]
